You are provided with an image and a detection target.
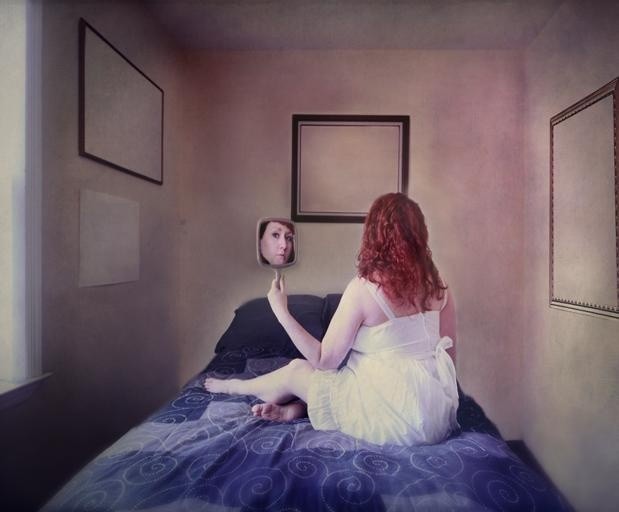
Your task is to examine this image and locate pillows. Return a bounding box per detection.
[213,293,325,354]
[320,293,345,336]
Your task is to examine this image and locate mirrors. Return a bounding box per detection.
[255,216,299,268]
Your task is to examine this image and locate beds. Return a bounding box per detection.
[36,292,578,510]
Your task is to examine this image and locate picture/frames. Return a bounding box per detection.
[547,74,618,319]
[76,16,166,189]
[290,113,412,224]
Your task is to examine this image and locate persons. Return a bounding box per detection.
[260,221,295,265]
[202,191,461,447]
